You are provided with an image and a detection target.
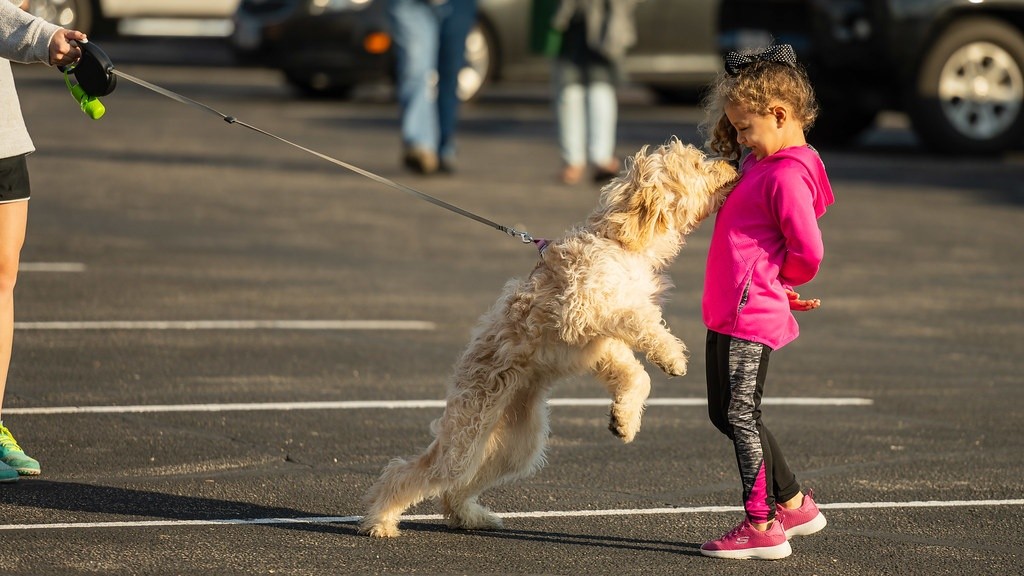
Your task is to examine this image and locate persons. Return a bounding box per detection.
[699,44,835,560]
[528,0,639,186]
[385,0,478,176]
[0,0,85,479]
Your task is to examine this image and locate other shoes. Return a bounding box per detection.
[403,146,458,175]
[552,155,627,185]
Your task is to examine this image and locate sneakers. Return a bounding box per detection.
[775,487,828,539]
[0,420,42,482]
[700,515,793,560]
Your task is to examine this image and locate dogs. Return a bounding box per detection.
[356,137,742,537]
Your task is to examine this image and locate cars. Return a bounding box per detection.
[22,0,1024,157]
